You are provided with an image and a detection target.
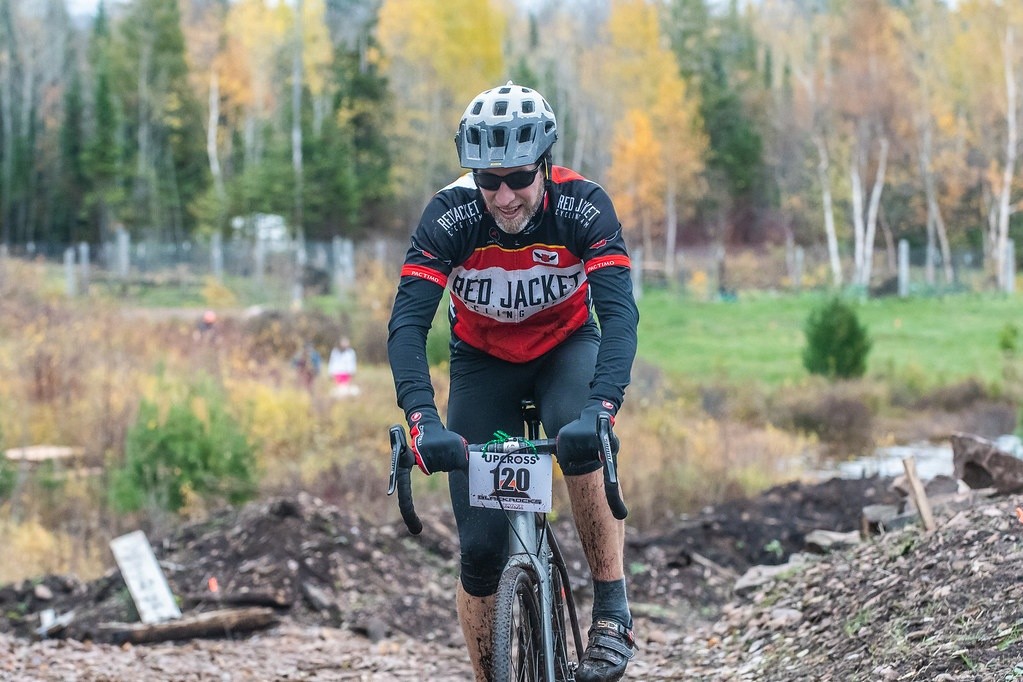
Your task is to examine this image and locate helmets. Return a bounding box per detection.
[454,80,558,168]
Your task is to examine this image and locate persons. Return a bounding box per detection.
[386,82,639,682]
[330,336,361,398]
[295,341,321,385]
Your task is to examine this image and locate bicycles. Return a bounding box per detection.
[385,398,632,682]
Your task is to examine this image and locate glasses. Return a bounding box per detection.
[473,162,543,191]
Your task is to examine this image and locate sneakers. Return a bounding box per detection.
[576,609,639,682]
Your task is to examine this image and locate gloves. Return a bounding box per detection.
[555,399,619,467]
[406,406,470,476]
